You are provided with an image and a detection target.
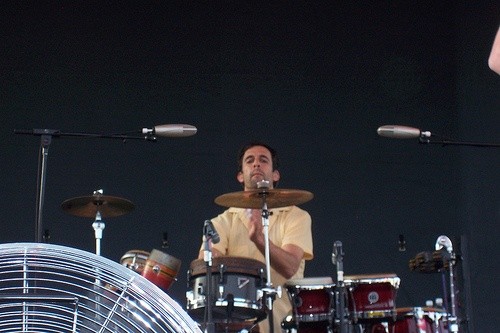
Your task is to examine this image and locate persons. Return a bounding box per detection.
[197,136,314,333]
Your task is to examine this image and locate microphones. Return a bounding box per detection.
[204,220,220,244]
[377,125,432,140]
[332,241,345,264]
[133,124,197,138]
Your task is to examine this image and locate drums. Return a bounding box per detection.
[284,273,395,321]
[186,256,275,333]
[98,248,181,317]
[363,307,455,333]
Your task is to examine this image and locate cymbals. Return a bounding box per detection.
[409,250,465,274]
[214,187,313,210]
[61,195,134,219]
